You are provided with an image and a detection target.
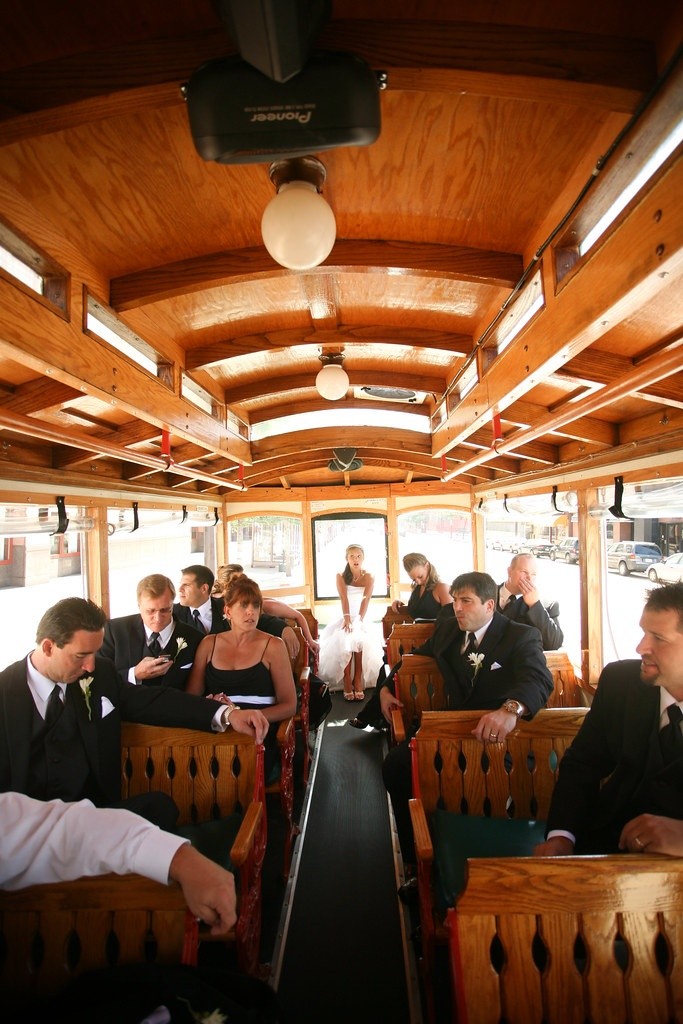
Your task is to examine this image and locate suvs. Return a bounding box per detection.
[607,541,662,576]
[549,537,580,564]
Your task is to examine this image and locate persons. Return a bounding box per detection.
[377,572,554,902]
[184,572,297,786]
[0,791,282,1024]
[319,544,384,701]
[214,558,319,663]
[0,596,268,831]
[492,582,683,972]
[97,573,207,691]
[349,553,454,737]
[173,564,300,660]
[494,552,564,651]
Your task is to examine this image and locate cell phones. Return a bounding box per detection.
[158,655,172,665]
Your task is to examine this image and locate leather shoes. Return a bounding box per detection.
[398,877,419,906]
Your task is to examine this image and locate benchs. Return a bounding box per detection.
[0,605,683,1024]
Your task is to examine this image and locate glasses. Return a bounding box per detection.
[141,605,174,616]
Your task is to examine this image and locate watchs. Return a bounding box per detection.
[503,699,523,719]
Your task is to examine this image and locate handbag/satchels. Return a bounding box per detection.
[307,673,332,731]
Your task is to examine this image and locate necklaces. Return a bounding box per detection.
[353,570,362,583]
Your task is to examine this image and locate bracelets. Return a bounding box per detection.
[223,705,240,725]
[343,614,350,616]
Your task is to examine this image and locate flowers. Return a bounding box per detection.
[468,652,485,688]
[173,637,188,664]
[79,676,95,721]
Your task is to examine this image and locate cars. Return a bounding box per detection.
[492,540,553,558]
[646,552,683,585]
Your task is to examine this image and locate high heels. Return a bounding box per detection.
[350,680,365,701]
[349,716,376,728]
[343,678,354,701]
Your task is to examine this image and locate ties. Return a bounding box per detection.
[463,632,478,668]
[503,594,516,613]
[44,685,64,732]
[658,704,683,770]
[193,610,207,634]
[147,632,162,657]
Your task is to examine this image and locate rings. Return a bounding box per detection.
[346,625,348,626]
[490,734,497,737]
[635,838,645,847]
